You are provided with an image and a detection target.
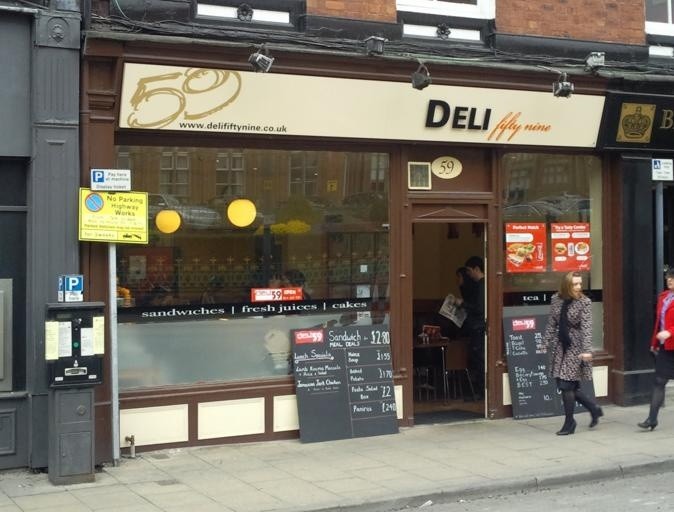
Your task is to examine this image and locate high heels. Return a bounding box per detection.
[589,406,603,427]
[556,419,577,436]
[637,418,658,431]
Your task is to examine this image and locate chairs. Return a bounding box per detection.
[413,337,479,402]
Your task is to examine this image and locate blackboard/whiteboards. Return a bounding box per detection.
[289,324,399,443]
[502,314,595,421]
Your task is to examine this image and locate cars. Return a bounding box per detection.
[505,192,590,222]
[209,195,263,230]
[149,193,221,230]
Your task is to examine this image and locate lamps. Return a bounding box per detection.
[585,52,607,74]
[363,36,389,58]
[412,58,432,90]
[553,71,575,96]
[227,198,257,228]
[155,207,181,233]
[248,40,274,74]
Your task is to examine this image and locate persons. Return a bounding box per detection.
[455,265,478,305]
[457,254,486,402]
[284,268,312,301]
[141,286,168,304]
[540,270,604,435]
[637,265,674,431]
